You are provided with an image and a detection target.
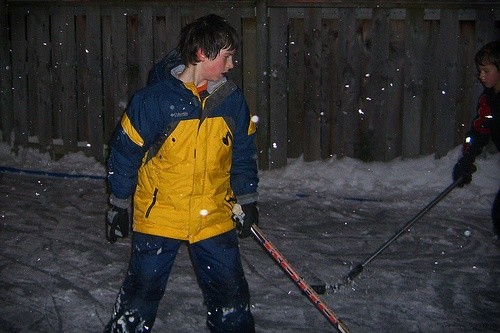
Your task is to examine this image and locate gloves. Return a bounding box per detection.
[231,206,258,238]
[105,205,129,244]
[453,154,476,187]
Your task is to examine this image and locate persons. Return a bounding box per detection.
[453,40,500,242]
[103,13,260,333]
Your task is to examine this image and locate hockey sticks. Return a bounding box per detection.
[232,204,350,333]
[309,165,478,296]
